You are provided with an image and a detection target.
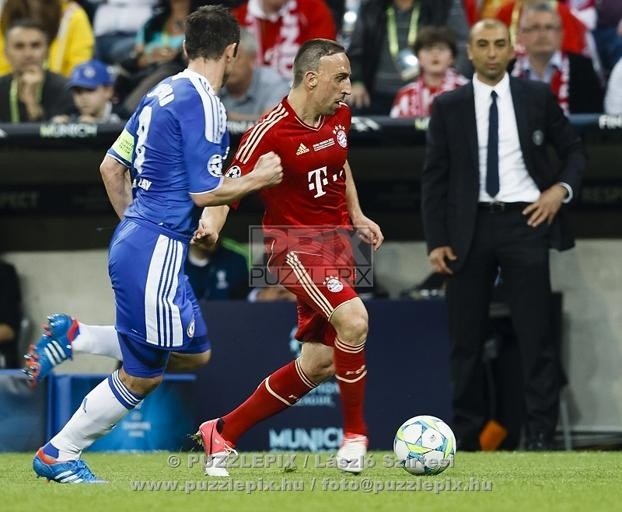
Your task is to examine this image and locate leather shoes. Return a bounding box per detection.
[456,434,477,451]
[524,417,548,451]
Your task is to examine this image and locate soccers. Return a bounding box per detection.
[394,416,456,476]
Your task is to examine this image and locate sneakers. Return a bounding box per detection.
[33,447,112,485]
[18,313,80,390]
[335,436,369,472]
[195,416,238,476]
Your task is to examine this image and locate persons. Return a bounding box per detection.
[244,232,389,305]
[0,0,622,131]
[20,3,284,485]
[1,256,24,374]
[401,266,510,303]
[188,36,387,480]
[178,211,249,300]
[419,16,594,453]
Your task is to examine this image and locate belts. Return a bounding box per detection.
[474,202,534,216]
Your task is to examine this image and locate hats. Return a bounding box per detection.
[63,58,111,89]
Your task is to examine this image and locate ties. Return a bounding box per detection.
[485,90,500,198]
